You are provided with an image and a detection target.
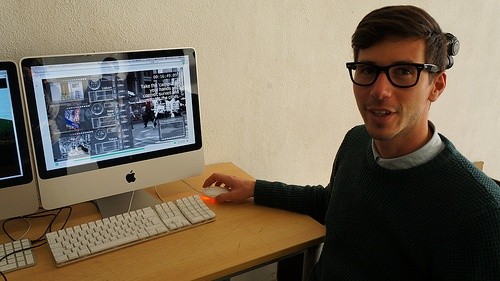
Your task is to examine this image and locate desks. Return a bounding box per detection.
[0,162,325,281]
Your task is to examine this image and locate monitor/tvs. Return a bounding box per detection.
[19,47,205,211]
[0,59,40,221]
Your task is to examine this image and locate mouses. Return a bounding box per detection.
[202,186,233,201]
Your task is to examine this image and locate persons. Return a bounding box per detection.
[80,58,135,154]
[22,59,64,160]
[144,99,157,128]
[203,5,500,281]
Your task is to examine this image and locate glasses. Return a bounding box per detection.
[346,62,439,88]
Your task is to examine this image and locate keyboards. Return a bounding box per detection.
[45,194,217,269]
[0,238,38,275]
